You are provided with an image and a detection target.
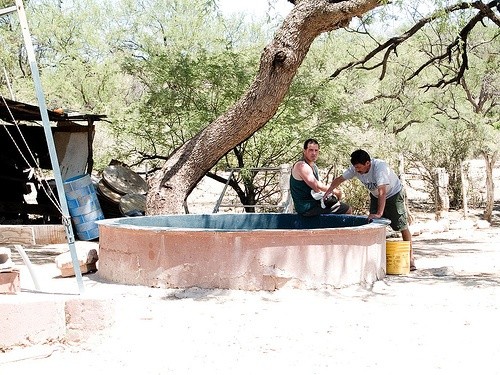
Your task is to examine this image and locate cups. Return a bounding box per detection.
[323,196,338,209]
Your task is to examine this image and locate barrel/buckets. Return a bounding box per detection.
[386,239,411,274]
[62,173,105,241]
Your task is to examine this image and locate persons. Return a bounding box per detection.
[289,138,353,216]
[323,149,418,271]
